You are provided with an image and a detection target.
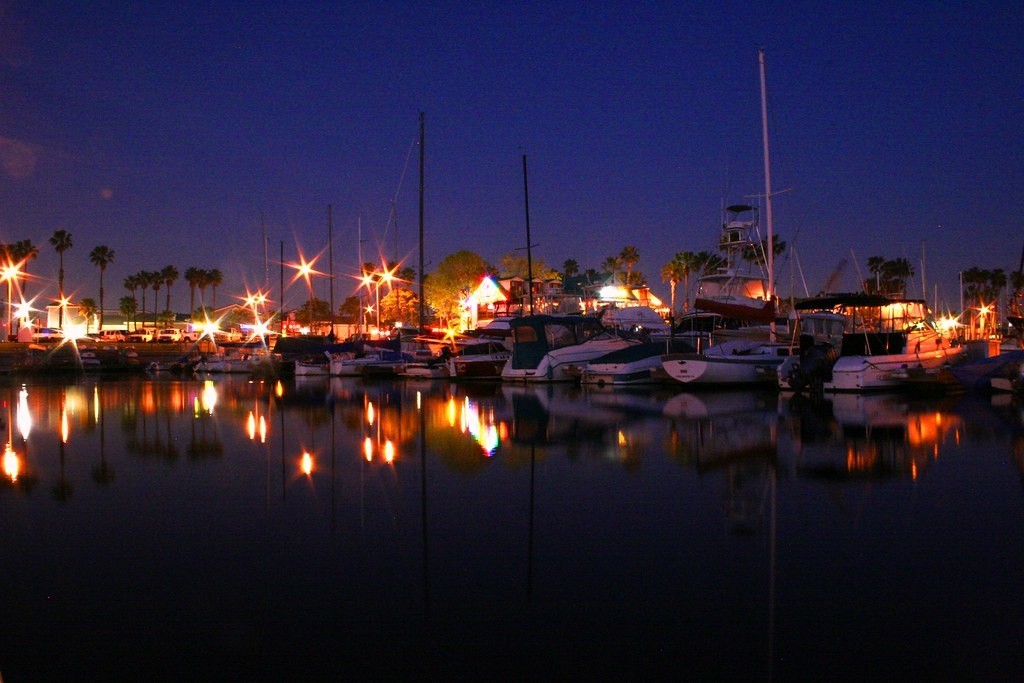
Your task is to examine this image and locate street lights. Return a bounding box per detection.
[2,262,20,341]
[298,260,315,336]
[376,271,393,334]
[254,293,268,339]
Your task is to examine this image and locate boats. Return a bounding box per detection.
[81,46,1023,392]
